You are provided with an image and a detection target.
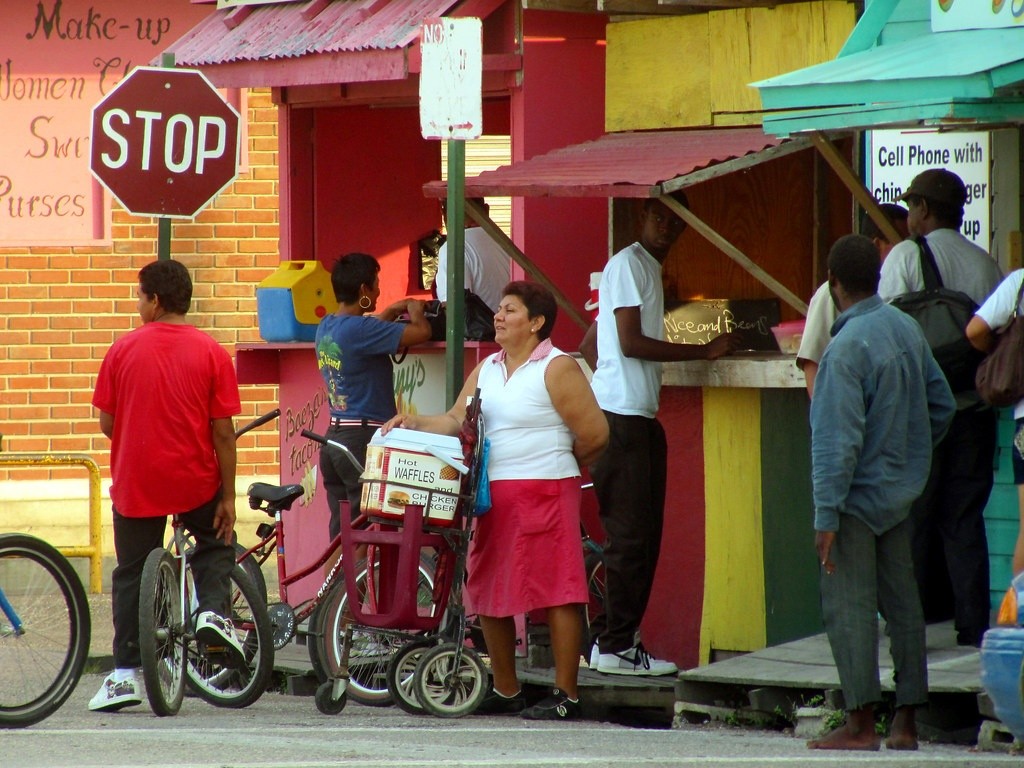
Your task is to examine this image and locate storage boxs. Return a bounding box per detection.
[358,427,469,528]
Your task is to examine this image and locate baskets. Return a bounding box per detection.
[357,477,476,539]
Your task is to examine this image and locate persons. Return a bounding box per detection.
[579,186,744,676]
[380,281,610,723]
[315,253,434,660]
[791,167,1024,751]
[435,187,513,344]
[87,259,246,712]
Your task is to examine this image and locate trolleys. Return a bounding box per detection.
[313,411,489,720]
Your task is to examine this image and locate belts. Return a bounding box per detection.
[329,416,384,427]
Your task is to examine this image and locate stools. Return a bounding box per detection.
[338,498,459,631]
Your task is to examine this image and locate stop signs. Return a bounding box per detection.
[87,65,243,222]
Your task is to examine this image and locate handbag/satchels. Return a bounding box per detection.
[437,289,495,341]
[889,231,999,403]
[391,299,446,364]
[975,278,1024,407]
[464,438,492,517]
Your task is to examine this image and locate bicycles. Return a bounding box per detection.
[138,409,282,717]
[155,430,453,703]
[1,532,94,730]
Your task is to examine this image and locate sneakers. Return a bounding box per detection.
[339,630,389,658]
[597,628,678,676]
[588,636,600,670]
[195,611,246,670]
[88,672,142,713]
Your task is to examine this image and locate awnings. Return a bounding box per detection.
[747,28,1024,140]
[147,0,489,87]
[422,126,855,356]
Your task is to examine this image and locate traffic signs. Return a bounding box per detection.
[419,16,482,140]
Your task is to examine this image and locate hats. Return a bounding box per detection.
[893,168,967,207]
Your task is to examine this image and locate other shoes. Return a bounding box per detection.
[520,688,587,720]
[468,683,526,716]
[956,622,991,649]
